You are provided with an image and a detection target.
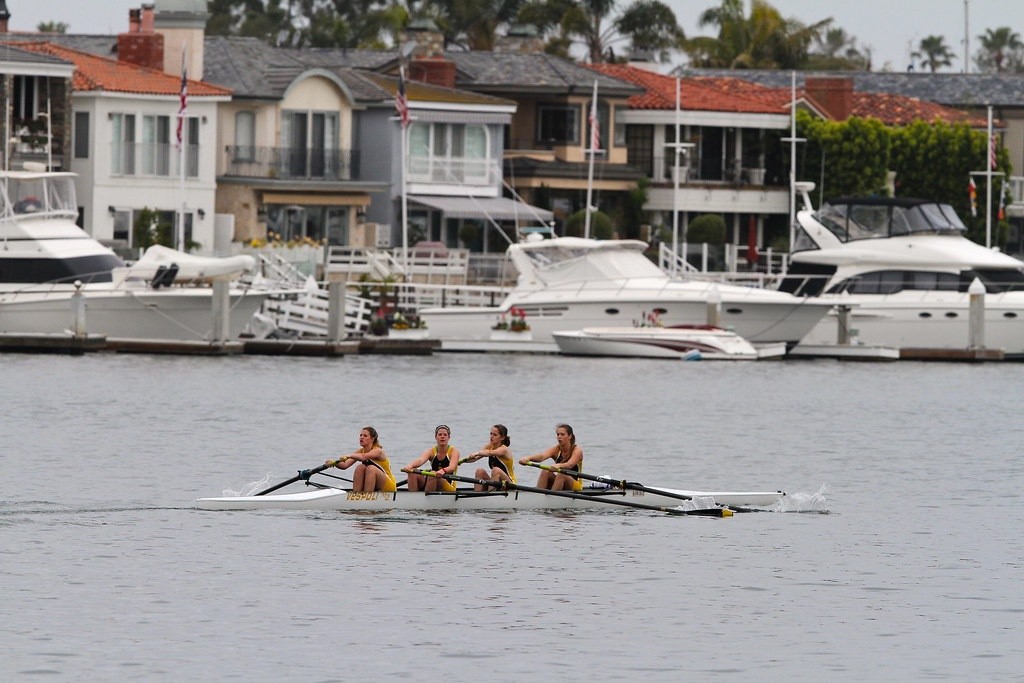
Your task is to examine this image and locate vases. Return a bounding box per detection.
[372,327,430,338]
[490,330,531,341]
[669,165,689,182]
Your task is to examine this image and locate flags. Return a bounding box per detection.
[176,46,189,149]
[590,86,600,151]
[989,114,997,169]
[394,58,410,130]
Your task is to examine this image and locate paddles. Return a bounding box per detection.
[252,456,349,497]
[521,457,832,518]
[394,450,485,489]
[399,467,735,520]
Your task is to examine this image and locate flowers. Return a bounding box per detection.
[371,310,428,329]
[491,307,530,333]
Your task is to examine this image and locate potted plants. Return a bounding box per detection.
[745,156,766,184]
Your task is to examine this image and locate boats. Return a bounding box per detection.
[197,475,788,510]
[0,43,859,356]
[551,312,759,360]
[764,180,1024,362]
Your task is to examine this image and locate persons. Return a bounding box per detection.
[325,426,398,492]
[465,424,517,492]
[404,425,460,492]
[519,423,584,492]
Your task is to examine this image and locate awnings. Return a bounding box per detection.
[406,193,553,220]
